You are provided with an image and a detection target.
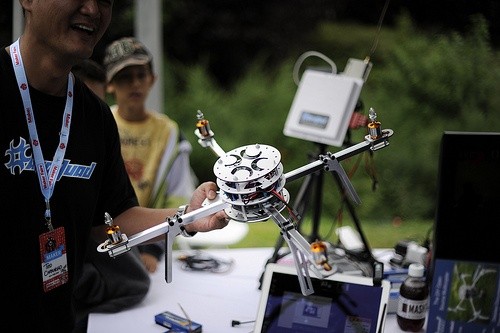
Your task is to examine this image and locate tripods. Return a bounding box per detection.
[258,142,376,293]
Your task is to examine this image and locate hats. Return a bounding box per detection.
[103,36,154,82]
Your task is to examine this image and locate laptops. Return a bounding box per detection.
[254,263,392,333]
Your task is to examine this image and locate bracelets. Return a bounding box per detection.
[176,205,197,238]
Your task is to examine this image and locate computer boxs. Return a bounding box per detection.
[428,129,500,291]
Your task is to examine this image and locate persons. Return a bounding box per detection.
[0,0,231,332]
[104,37,195,272]
